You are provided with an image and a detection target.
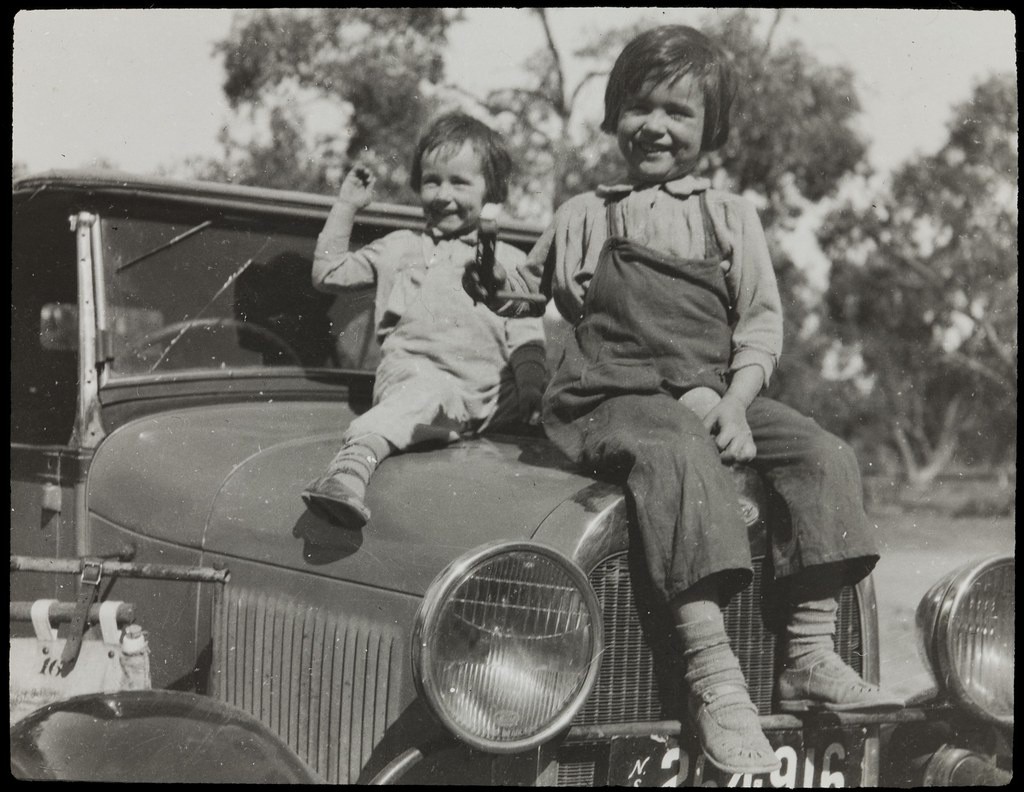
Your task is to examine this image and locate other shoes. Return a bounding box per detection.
[687,683,783,774]
[777,652,905,712]
[301,467,371,529]
[517,398,545,438]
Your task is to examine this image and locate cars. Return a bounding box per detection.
[11,175,1016,787]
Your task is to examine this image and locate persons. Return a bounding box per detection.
[302,109,551,527]
[462,21,906,776]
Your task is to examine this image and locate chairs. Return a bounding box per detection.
[26,270,183,439]
